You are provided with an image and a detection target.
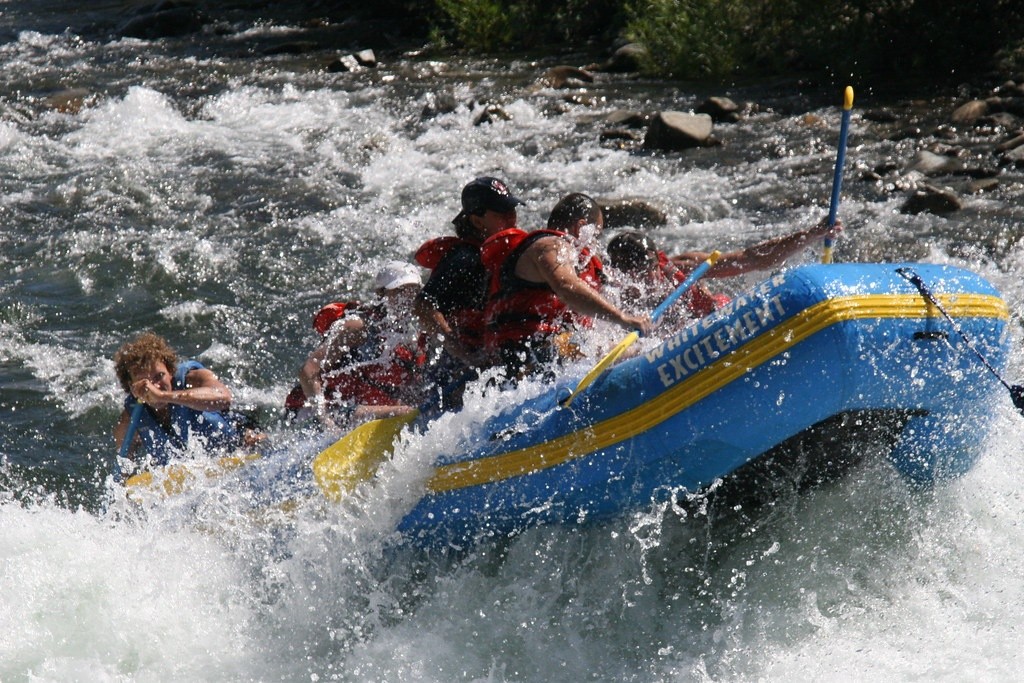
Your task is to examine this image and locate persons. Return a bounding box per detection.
[114,330,239,473]
[287,177,845,425]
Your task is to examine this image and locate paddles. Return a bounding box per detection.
[114,384,148,483]
[557,248,726,411]
[817,84,856,266]
[124,444,287,509]
[309,367,482,498]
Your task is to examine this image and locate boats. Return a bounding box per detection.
[131,84,1012,583]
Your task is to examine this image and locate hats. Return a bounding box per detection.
[313,301,357,333]
[607,230,657,268]
[452,177,527,225]
[376,261,422,289]
